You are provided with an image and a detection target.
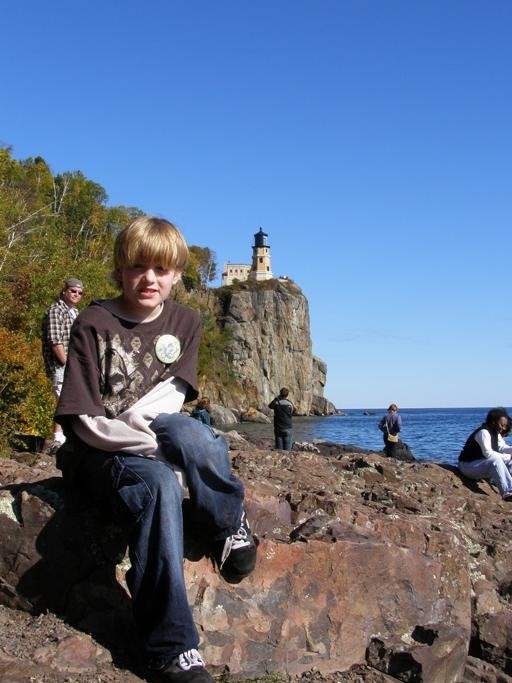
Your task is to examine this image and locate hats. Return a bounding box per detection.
[65,280,83,288]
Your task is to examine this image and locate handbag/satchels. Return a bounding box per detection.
[387,433,398,443]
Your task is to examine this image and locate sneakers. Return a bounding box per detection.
[503,492,512,499]
[146,648,216,683]
[47,440,61,455]
[211,499,257,579]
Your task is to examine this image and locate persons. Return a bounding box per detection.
[55,215,256,682]
[378,405,402,457]
[42,277,84,445]
[269,387,295,451]
[458,410,512,501]
[191,398,212,425]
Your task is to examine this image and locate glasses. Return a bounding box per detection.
[67,288,83,295]
